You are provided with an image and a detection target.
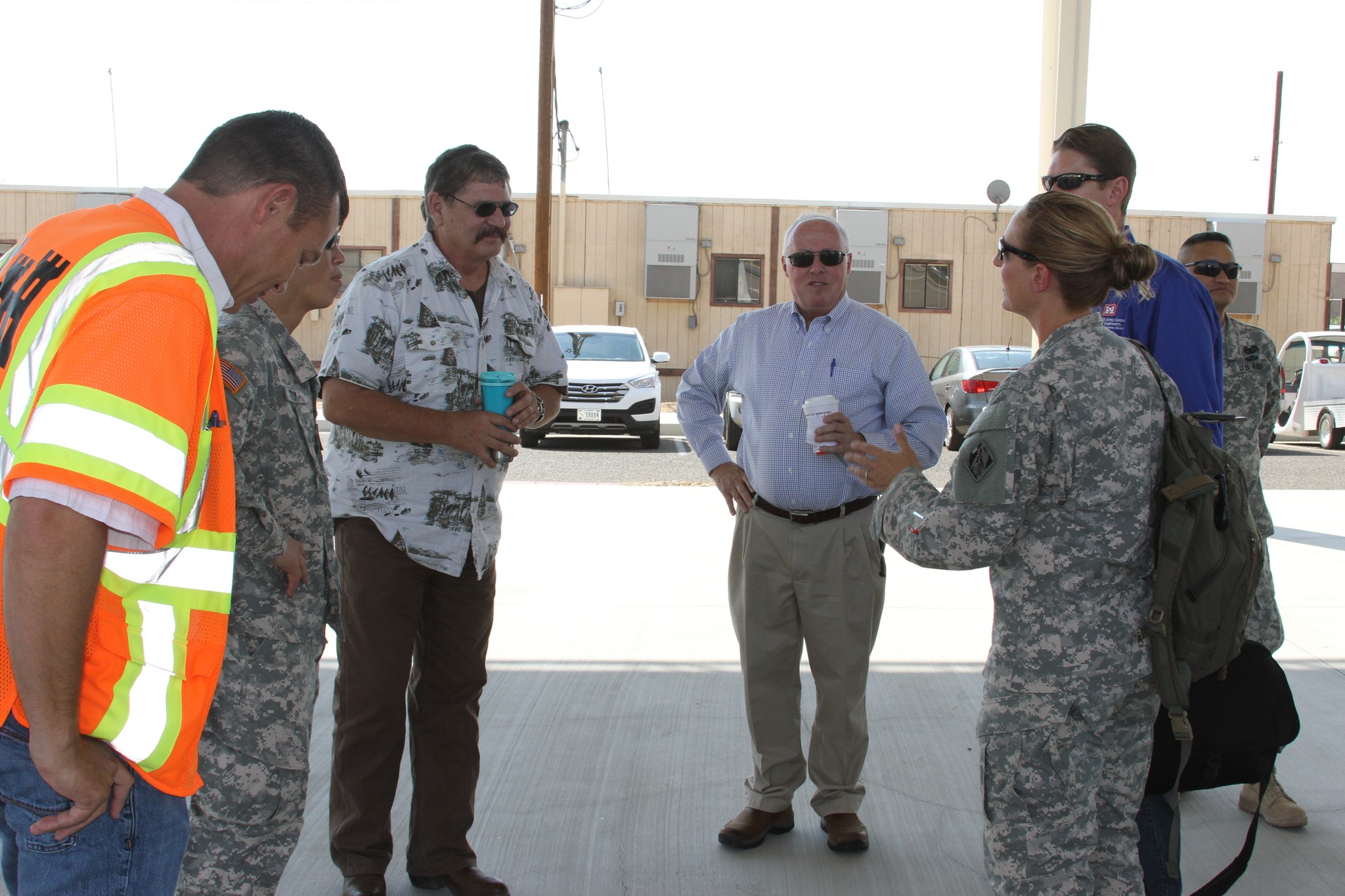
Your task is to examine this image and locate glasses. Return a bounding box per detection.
[784,250,852,268]
[997,237,1040,263]
[437,191,519,217]
[325,231,340,251]
[1040,172,1122,192]
[1183,259,1243,280]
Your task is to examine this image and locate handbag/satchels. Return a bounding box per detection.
[1144,637,1301,797]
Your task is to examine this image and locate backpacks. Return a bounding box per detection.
[1125,335,1265,742]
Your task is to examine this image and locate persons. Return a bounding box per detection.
[171,224,345,896]
[1161,229,1311,829]
[676,210,947,853]
[844,194,1188,896]
[1032,124,1230,894]
[319,145,570,896]
[2,107,348,896]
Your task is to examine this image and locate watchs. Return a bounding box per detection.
[533,392,546,423]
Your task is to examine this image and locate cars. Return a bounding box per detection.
[1270,331,1345,448]
[928,336,1033,451]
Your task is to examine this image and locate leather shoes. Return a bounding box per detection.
[342,874,387,896]
[408,866,510,896]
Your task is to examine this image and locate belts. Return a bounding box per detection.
[744,482,879,525]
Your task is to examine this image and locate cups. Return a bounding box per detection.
[802,395,840,454]
[479,371,517,463]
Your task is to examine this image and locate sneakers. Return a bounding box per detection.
[820,812,870,852]
[717,802,795,849]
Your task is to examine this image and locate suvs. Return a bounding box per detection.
[518,324,672,450]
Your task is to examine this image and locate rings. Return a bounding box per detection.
[528,406,534,414]
[864,469,871,483]
